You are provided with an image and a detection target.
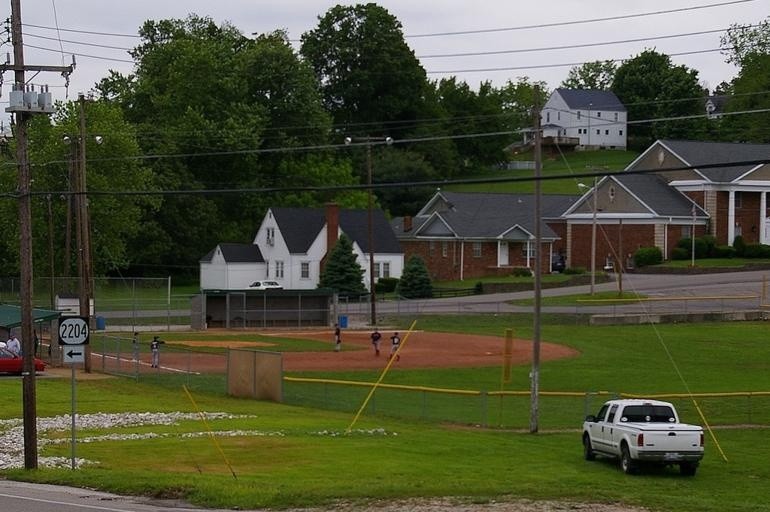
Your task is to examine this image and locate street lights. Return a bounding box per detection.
[575,175,599,295]
[344,133,396,326]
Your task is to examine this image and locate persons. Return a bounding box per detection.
[133,332,138,362]
[390,332,400,361]
[335,324,341,351]
[151,336,165,368]
[371,328,381,355]
[7,333,21,354]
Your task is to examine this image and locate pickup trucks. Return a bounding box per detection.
[581,398,706,477]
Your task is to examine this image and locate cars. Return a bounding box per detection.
[246,279,284,289]
